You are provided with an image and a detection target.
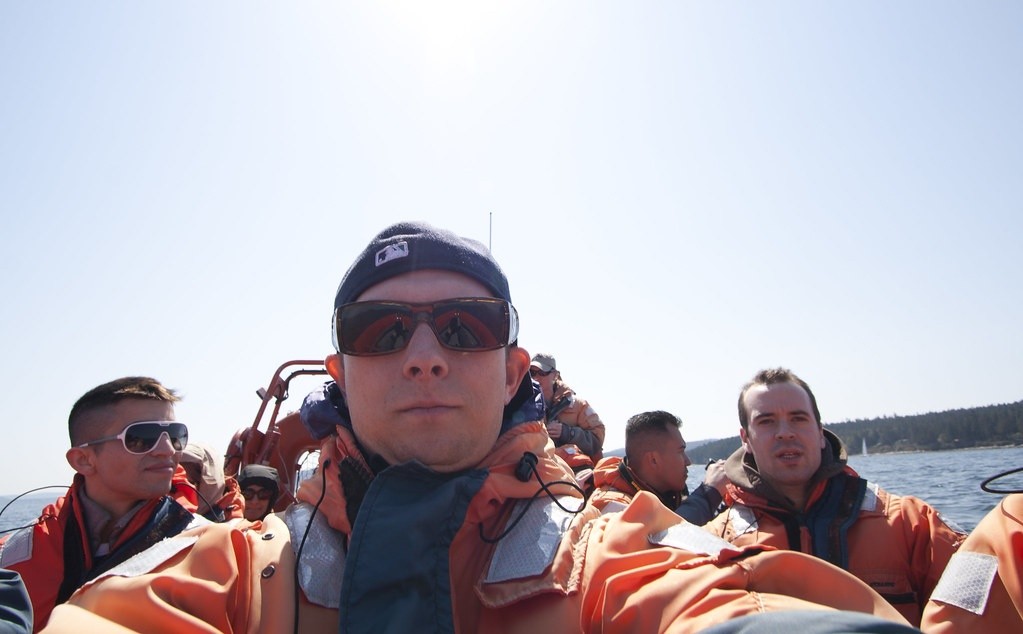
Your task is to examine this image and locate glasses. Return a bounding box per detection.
[243,487,273,500]
[332,297,519,359]
[531,371,551,376]
[78,421,188,454]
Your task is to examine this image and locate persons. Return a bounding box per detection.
[528,351,604,500]
[920,494,1023,634]
[586,411,730,528]
[236,464,281,523]
[699,368,970,627]
[34,221,923,634]
[0,377,215,634]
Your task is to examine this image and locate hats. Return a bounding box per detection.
[334,222,512,308]
[531,353,556,372]
